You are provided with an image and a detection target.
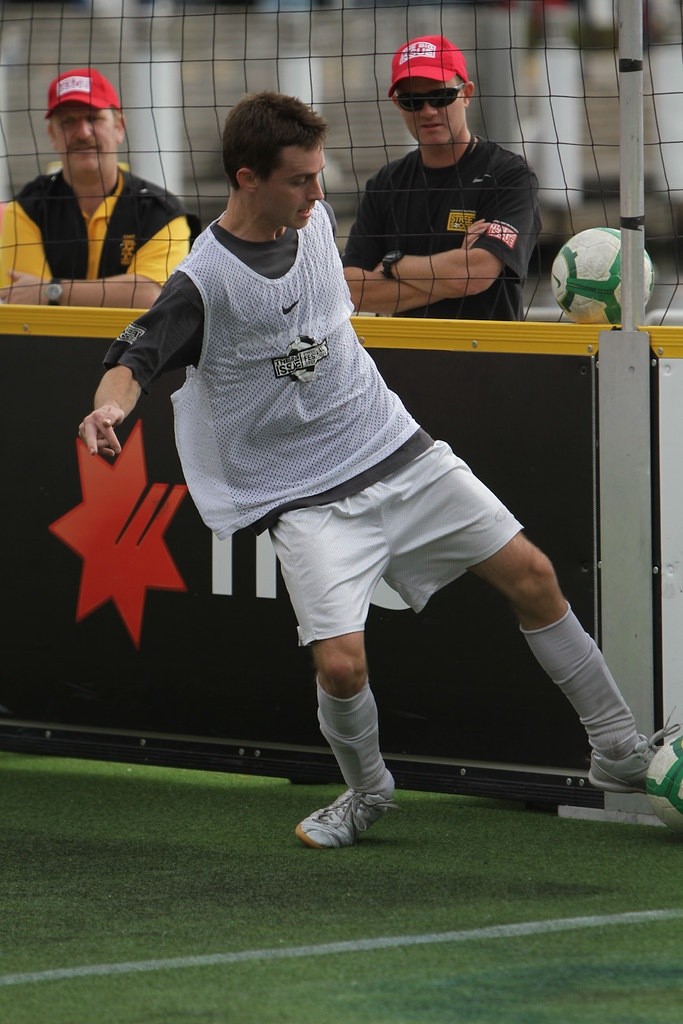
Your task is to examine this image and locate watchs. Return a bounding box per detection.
[380,251,404,279]
[45,278,63,305]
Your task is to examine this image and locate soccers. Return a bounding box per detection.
[550,227,655,324]
[644,734,683,834]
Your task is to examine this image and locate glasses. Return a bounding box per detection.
[399,83,466,111]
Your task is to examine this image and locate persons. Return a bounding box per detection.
[0,68,191,308]
[343,36,543,322]
[78,88,681,846]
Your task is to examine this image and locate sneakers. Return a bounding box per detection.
[295,789,402,848]
[588,705,682,794]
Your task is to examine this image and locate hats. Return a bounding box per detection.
[45,68,121,118]
[388,36,469,97]
